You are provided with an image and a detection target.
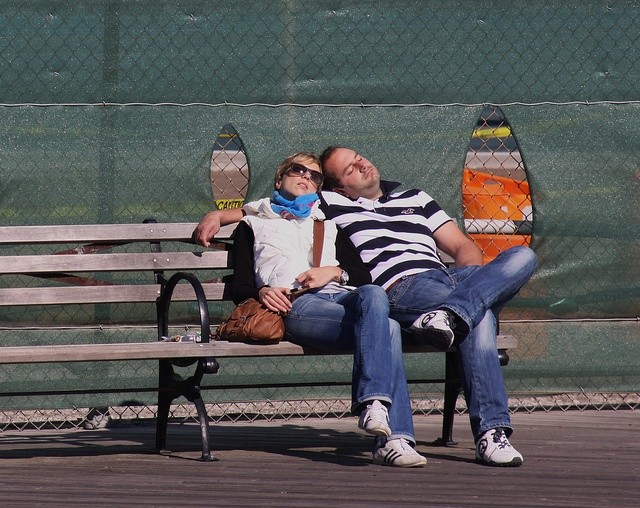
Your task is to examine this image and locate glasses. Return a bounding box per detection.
[285,162,325,184]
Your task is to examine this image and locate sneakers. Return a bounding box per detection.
[372,436,428,468]
[357,398,392,437]
[410,309,455,351]
[475,426,524,466]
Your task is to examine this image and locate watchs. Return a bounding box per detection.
[340,269,349,286]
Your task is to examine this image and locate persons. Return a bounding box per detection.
[194,146,540,468]
[227,151,428,467]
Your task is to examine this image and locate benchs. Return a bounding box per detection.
[0,222,518,461]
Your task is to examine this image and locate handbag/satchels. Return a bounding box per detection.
[215,296,291,345]
